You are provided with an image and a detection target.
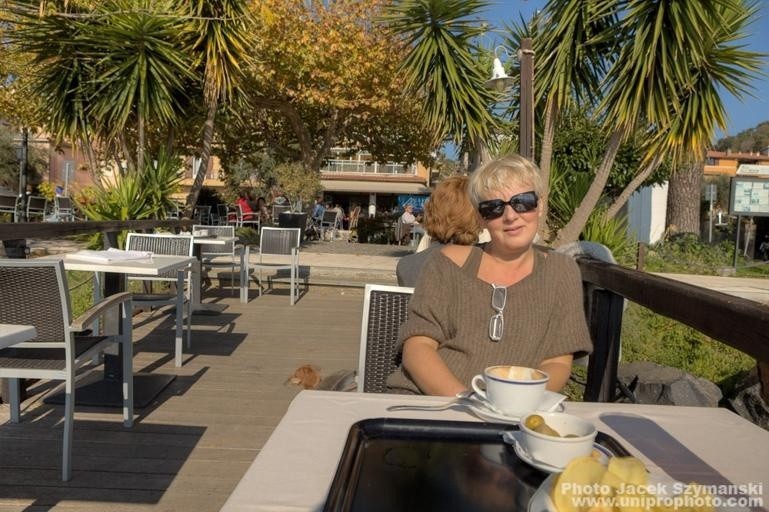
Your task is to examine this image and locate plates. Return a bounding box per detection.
[510,439,616,474]
[464,391,559,427]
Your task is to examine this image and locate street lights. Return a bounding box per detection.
[489,34,535,166]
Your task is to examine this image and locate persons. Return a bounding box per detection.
[235,188,290,230]
[25,184,36,221]
[314,201,361,227]
[386,152,594,394]
[396,177,486,288]
[401,204,424,236]
[760,235,769,261]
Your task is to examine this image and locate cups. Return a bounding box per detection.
[470,364,548,416]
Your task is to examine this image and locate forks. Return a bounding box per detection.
[385,397,485,411]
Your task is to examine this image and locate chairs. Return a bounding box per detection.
[0,193,20,224]
[357,283,415,392]
[188,224,244,298]
[54,197,76,224]
[93,232,195,365]
[190,198,426,250]
[0,258,136,479]
[23,196,47,222]
[241,227,303,304]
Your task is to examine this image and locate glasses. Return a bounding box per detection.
[488,284,507,342]
[478,192,540,219]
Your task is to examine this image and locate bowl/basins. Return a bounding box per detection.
[518,411,598,467]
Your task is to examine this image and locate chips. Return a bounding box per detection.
[553,450,713,512]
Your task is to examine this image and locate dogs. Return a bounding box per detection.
[288,364,358,392]
